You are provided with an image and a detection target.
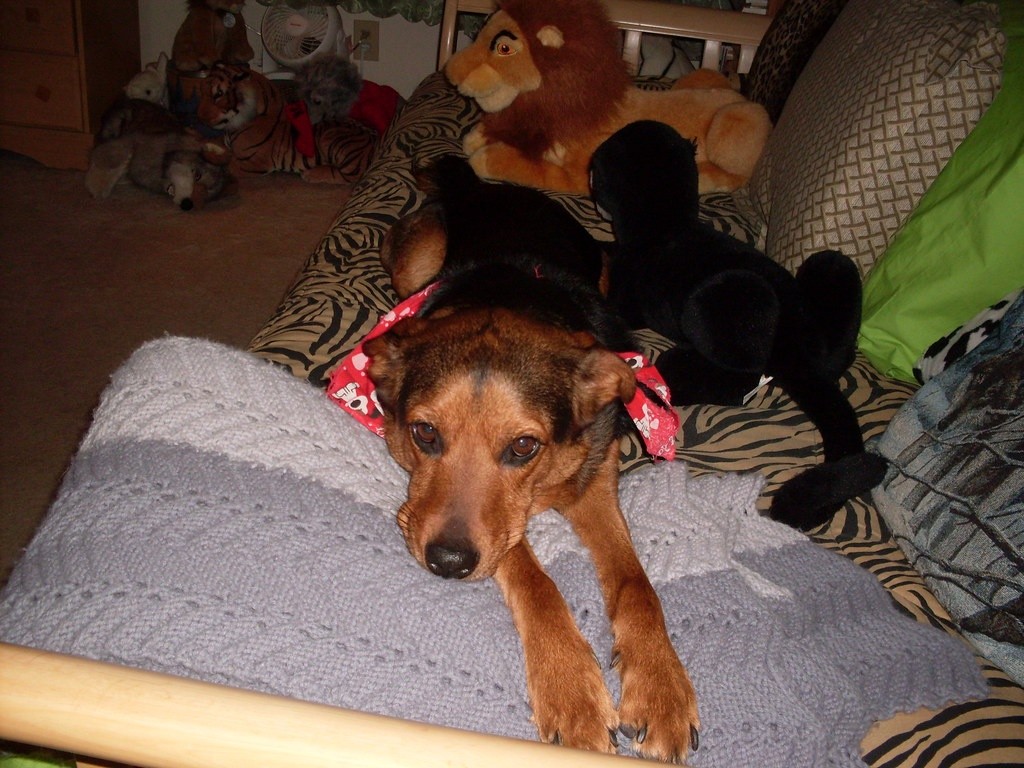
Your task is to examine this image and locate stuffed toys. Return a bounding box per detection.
[585,120,885,533]
[445,0,770,195]
[86,0,409,213]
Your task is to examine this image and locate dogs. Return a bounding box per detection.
[381,154,702,766]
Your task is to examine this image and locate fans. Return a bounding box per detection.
[258,3,339,66]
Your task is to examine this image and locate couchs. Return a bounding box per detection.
[0,0,1024,768]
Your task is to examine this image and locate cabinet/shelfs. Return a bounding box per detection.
[0,0,141,167]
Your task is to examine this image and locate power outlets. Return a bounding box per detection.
[352,19,381,62]
